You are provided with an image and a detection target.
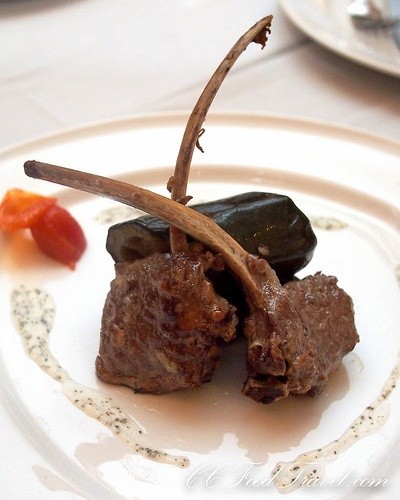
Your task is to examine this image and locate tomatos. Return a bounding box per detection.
[1,187,86,269]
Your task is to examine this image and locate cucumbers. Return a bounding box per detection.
[104,191,319,300]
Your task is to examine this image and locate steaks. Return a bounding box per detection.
[93,15,277,395]
[24,159,361,405]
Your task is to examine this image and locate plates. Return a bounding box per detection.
[0,110,400,499]
[276,0,399,79]
